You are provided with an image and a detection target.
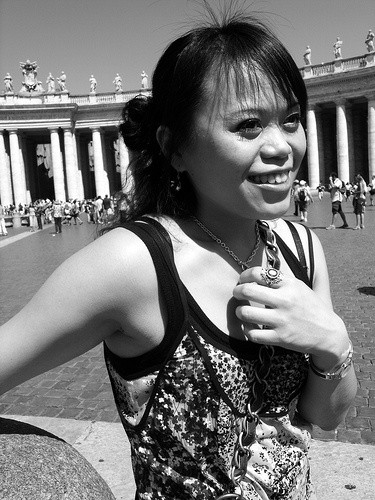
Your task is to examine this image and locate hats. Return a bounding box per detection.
[300,180,307,185]
[293,179,299,183]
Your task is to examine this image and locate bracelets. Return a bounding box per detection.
[309,339,353,381]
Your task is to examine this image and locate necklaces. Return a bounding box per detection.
[188,215,260,273]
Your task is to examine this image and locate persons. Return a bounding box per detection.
[89,75,97,93]
[0,0,358,500]
[0,194,129,237]
[3,72,12,91]
[293,172,375,230]
[112,73,124,92]
[334,37,343,59]
[57,71,67,91]
[365,30,374,52]
[304,45,312,65]
[141,71,148,88]
[47,73,55,92]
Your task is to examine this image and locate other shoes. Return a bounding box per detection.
[326,225,335,230]
[361,224,364,228]
[340,224,348,228]
[294,213,298,216]
[300,218,304,221]
[353,226,360,230]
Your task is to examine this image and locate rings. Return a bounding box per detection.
[261,266,284,287]
